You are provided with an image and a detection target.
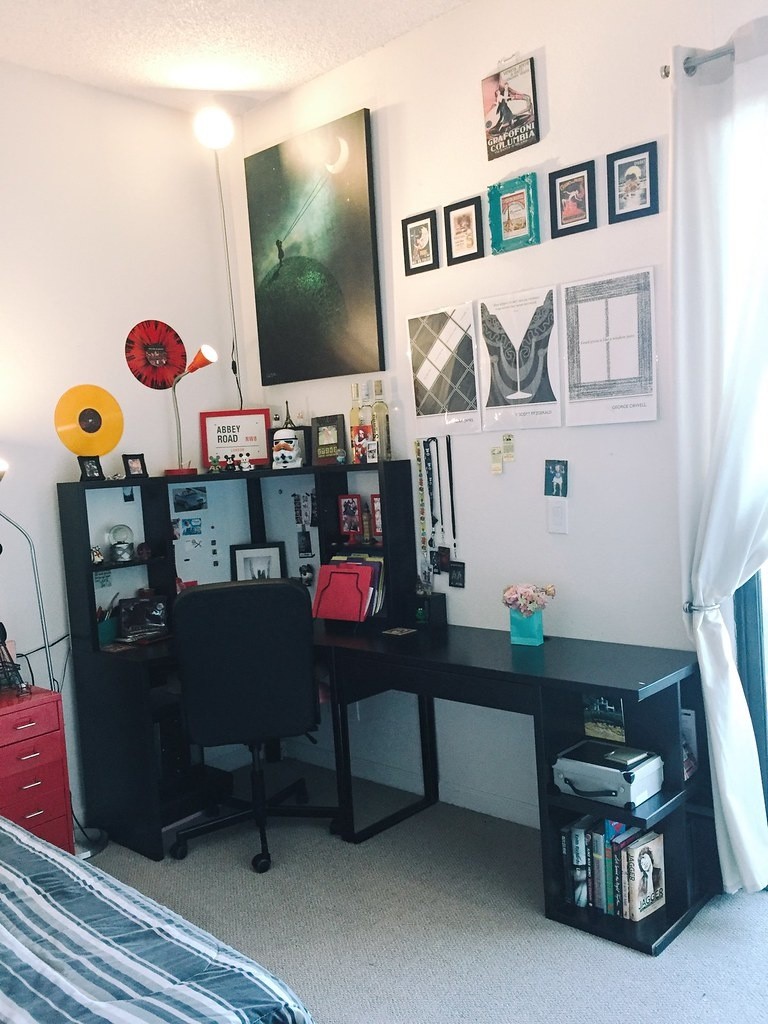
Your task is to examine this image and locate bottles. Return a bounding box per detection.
[359,381,372,426]
[372,381,392,462]
[349,382,361,463]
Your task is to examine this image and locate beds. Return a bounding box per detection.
[0,815,315,1024]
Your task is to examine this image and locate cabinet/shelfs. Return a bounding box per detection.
[0,684,76,858]
[335,623,725,956]
[56,459,432,863]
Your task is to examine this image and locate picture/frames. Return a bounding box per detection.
[229,541,288,581]
[487,173,540,256]
[366,441,378,463]
[371,493,384,547]
[122,453,148,479]
[549,160,597,239]
[606,141,660,224]
[402,210,439,276]
[443,196,485,266]
[337,494,363,543]
[77,455,106,481]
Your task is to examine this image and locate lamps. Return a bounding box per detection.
[164,344,219,477]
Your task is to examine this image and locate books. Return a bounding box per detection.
[560,815,666,922]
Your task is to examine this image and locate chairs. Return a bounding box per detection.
[170,581,343,873]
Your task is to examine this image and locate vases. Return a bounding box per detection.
[509,607,544,646]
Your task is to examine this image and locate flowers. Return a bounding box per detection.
[502,583,557,618]
